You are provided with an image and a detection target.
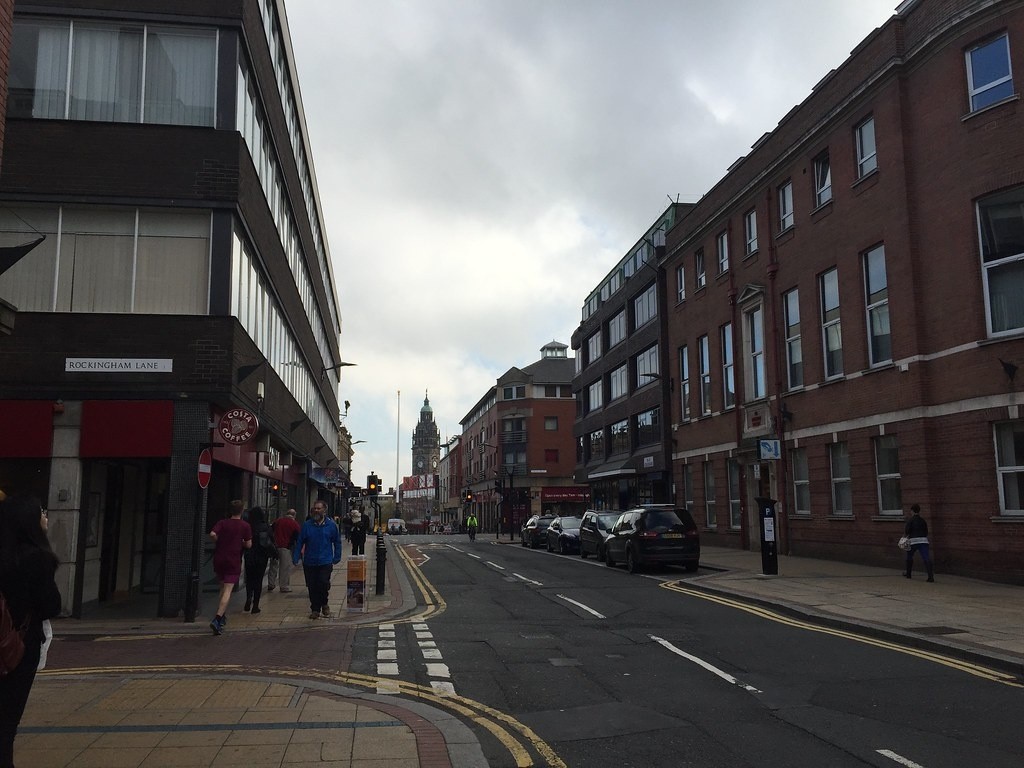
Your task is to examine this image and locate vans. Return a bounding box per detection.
[389,519,405,534]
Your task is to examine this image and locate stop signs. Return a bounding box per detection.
[197,449,211,489]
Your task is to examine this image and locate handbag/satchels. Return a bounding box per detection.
[898,533,912,551]
[0,592,34,674]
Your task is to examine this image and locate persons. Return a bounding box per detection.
[350,510,366,556]
[268,508,300,592]
[292,500,342,619]
[462,516,467,532]
[244,506,276,614]
[545,510,552,516]
[0,477,61,768]
[343,513,354,543]
[902,504,934,582]
[391,524,395,534]
[467,513,477,540]
[209,499,253,634]
[532,511,540,518]
[359,510,369,533]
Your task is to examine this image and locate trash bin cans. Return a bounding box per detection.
[346,555,366,612]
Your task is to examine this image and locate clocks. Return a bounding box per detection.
[433,460,437,468]
[417,459,424,469]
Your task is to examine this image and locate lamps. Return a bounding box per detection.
[320,363,356,380]
[482,442,498,454]
[639,371,675,391]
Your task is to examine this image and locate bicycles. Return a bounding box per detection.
[468,526,478,543]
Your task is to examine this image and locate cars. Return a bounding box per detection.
[546,517,583,555]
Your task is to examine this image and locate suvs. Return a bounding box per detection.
[579,509,626,563]
[520,514,559,549]
[603,508,700,572]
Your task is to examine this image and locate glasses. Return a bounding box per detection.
[42,508,48,518]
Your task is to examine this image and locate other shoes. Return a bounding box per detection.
[902,572,911,578]
[210,619,222,635]
[309,611,320,619]
[244,600,251,611]
[251,607,260,614]
[220,617,226,626]
[322,604,330,615]
[926,578,934,582]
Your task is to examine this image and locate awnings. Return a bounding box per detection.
[588,459,636,479]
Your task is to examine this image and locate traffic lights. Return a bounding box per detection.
[367,475,382,496]
[466,490,472,502]
[494,480,502,494]
